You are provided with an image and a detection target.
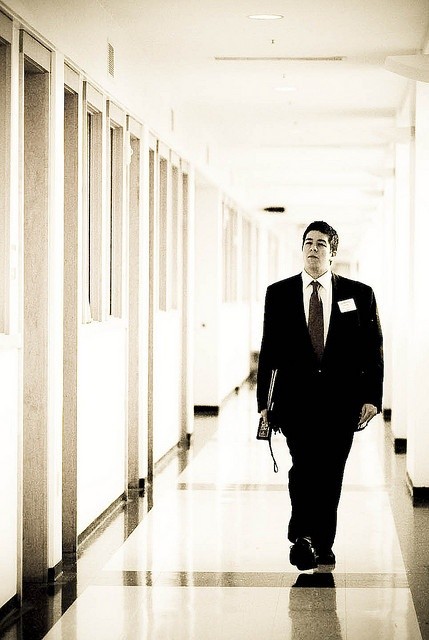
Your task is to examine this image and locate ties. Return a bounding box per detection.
[308,280,324,359]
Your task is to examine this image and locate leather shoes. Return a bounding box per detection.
[290,537,318,570]
[315,544,336,562]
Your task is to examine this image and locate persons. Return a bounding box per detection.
[255,220,385,573]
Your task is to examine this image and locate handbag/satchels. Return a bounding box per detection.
[261,368,292,429]
